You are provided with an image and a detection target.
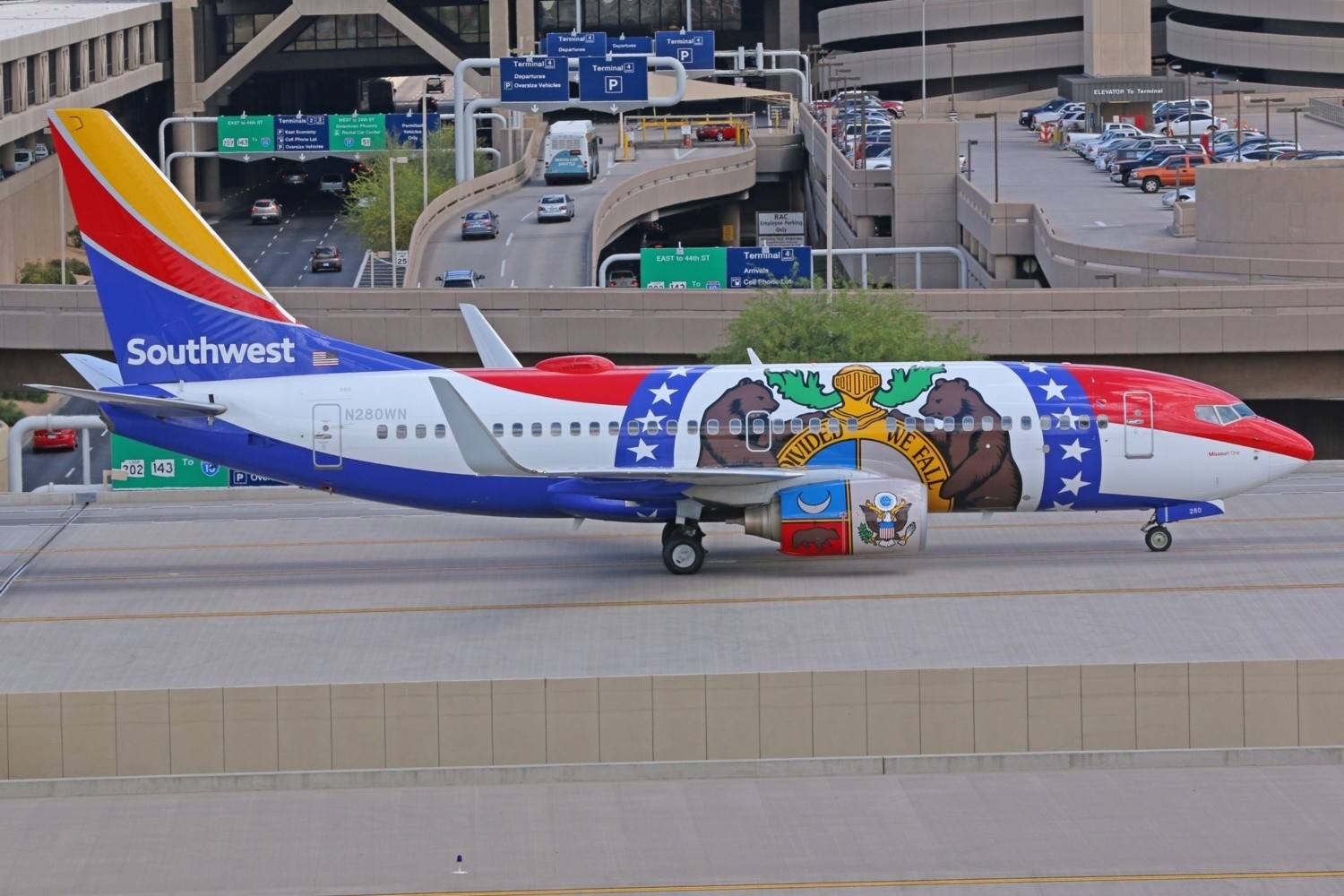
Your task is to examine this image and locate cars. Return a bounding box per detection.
[14,142,50,173]
[1210,63,1241,83]
[696,122,750,142]
[280,164,309,186]
[460,210,500,240]
[627,223,669,249]
[417,96,439,111]
[1161,186,1196,208]
[601,271,639,290]
[1019,97,1344,193]
[537,195,575,222]
[809,91,967,171]
[32,427,78,452]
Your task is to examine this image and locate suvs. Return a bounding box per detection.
[435,270,486,289]
[252,199,283,224]
[426,77,447,94]
[308,245,343,273]
[320,169,347,192]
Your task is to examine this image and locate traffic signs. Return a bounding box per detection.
[726,245,814,291]
[218,110,445,162]
[640,248,727,290]
[499,28,716,103]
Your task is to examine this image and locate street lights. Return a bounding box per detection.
[946,43,961,113]
[810,45,869,170]
[388,158,409,286]
[1151,63,1314,162]
[976,112,1000,203]
[967,139,978,182]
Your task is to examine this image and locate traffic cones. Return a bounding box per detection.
[684,134,691,146]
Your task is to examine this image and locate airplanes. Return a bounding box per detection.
[23,108,1317,576]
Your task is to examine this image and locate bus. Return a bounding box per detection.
[544,121,602,184]
[367,78,398,108]
